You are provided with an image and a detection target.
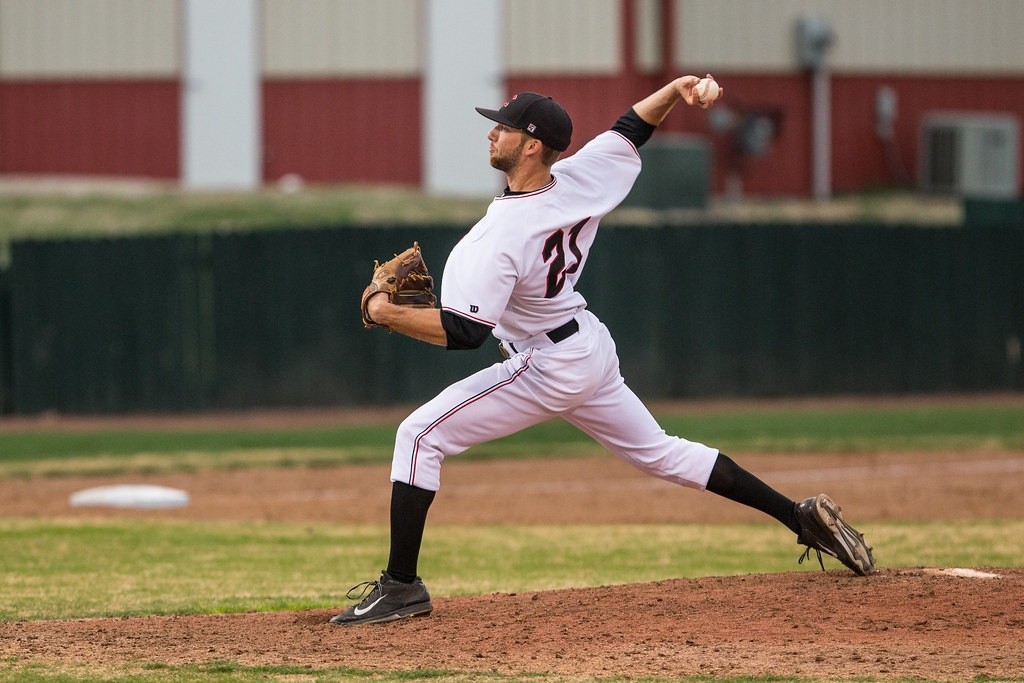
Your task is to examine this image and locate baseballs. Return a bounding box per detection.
[693,78,719,104]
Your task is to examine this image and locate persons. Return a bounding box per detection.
[329,74,877,626]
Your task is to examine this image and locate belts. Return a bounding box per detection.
[498,317,580,359]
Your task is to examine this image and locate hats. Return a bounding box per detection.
[476,92,573,151]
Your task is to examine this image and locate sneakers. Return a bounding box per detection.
[328,569,434,625]
[793,493,877,576]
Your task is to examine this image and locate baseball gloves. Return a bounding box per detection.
[359,246,439,330]
